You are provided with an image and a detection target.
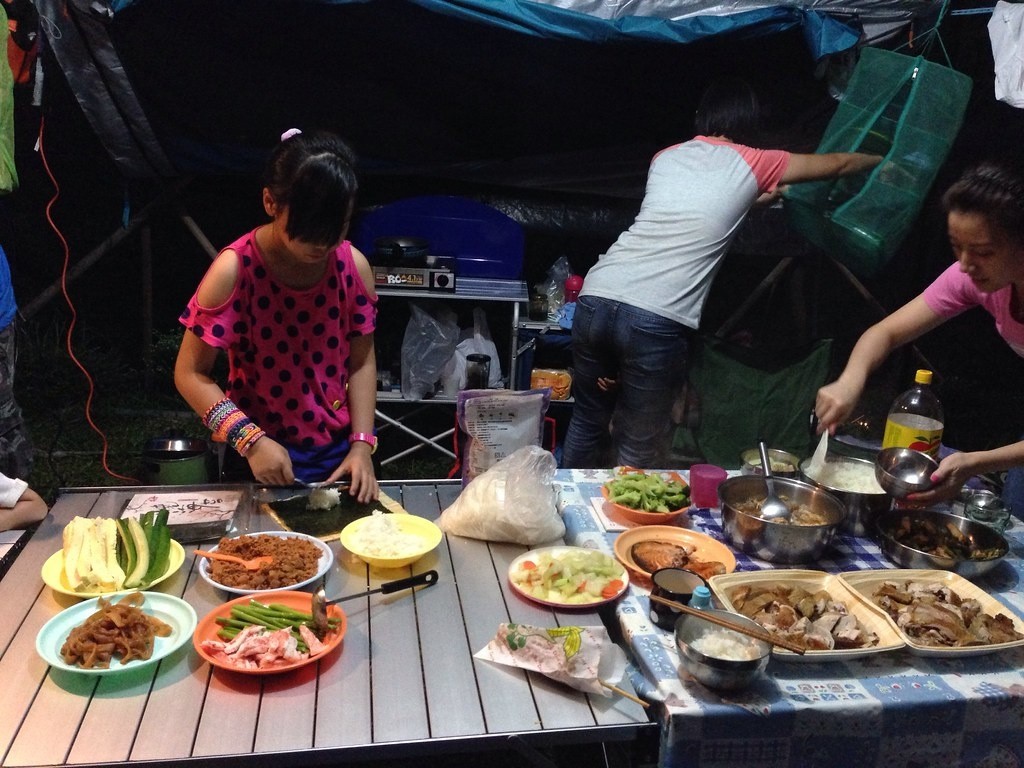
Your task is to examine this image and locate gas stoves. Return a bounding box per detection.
[365,256,457,291]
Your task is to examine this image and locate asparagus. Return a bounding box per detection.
[215,601,341,652]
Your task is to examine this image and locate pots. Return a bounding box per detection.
[140,431,213,486]
[377,236,429,260]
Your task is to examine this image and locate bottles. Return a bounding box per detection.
[882,370,944,463]
[564,275,583,304]
[529,294,548,321]
[689,586,712,611]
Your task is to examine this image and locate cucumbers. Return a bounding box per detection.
[116,508,171,588]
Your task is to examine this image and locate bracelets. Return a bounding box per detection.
[202,397,265,457]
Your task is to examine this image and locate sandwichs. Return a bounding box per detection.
[62,516,128,591]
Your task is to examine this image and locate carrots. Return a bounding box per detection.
[602,579,623,598]
[524,560,535,569]
[578,581,587,592]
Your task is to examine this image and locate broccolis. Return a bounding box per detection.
[606,473,692,515]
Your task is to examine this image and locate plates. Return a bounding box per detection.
[613,525,906,663]
[508,546,629,608]
[35,531,348,674]
[838,570,1024,658]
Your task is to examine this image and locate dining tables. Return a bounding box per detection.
[553,468,1024,767]
[1,479,658,767]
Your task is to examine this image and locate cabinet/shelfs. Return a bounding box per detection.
[375,277,575,468]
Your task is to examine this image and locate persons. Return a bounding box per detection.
[560,79,885,470]
[815,154,1024,510]
[174,129,379,504]
[0,472,48,532]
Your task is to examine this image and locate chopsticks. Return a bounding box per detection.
[649,594,806,655]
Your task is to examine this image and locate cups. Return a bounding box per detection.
[650,568,709,633]
[964,492,1011,530]
[690,464,726,508]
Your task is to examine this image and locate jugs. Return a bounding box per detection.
[467,354,491,390]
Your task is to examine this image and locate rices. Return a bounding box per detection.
[687,627,763,661]
[305,487,342,511]
[351,509,428,557]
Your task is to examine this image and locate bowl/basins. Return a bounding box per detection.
[340,514,442,569]
[876,508,1010,581]
[601,472,692,525]
[674,610,773,691]
[801,455,889,537]
[875,447,938,498]
[718,474,846,564]
[742,449,799,480]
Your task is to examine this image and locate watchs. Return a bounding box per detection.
[349,432,378,455]
